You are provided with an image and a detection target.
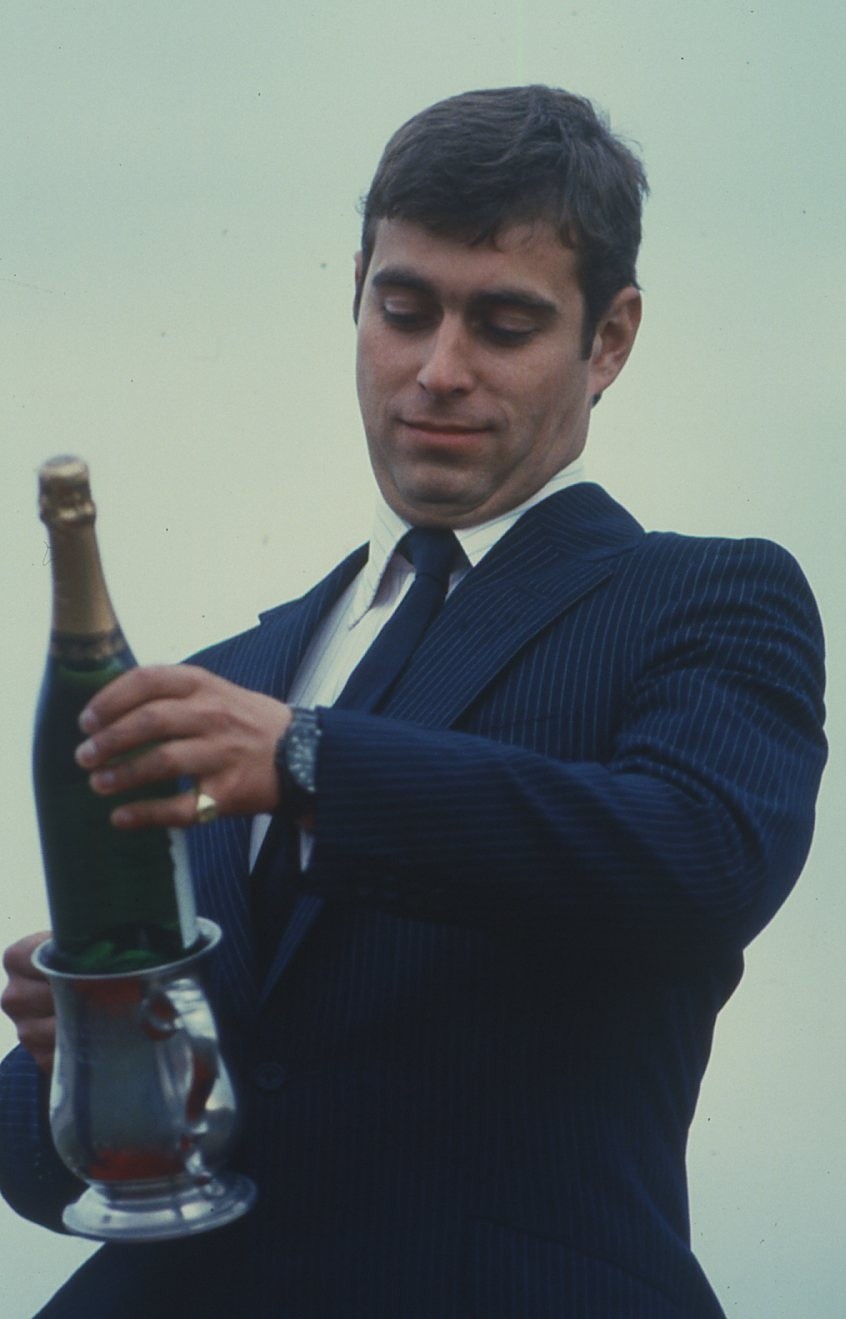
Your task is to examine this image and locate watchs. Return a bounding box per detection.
[269,706,320,818]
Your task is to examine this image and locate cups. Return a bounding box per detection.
[31,917,256,1241]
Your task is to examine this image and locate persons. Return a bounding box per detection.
[0,84,827,1319]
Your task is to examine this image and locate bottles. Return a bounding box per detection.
[31,457,206,974]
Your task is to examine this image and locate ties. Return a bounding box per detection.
[249,526,465,985]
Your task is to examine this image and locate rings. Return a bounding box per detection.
[196,795,217,822]
